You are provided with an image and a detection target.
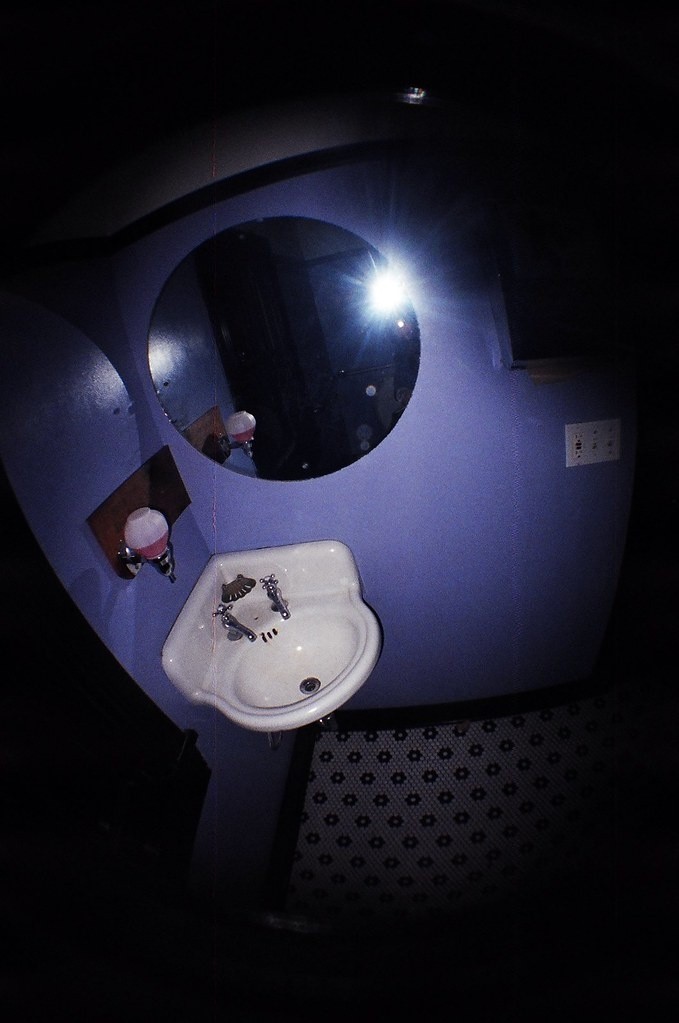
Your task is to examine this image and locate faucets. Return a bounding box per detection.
[211,602,258,643]
[259,573,292,621]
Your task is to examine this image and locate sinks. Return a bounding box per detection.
[161,538,382,733]
